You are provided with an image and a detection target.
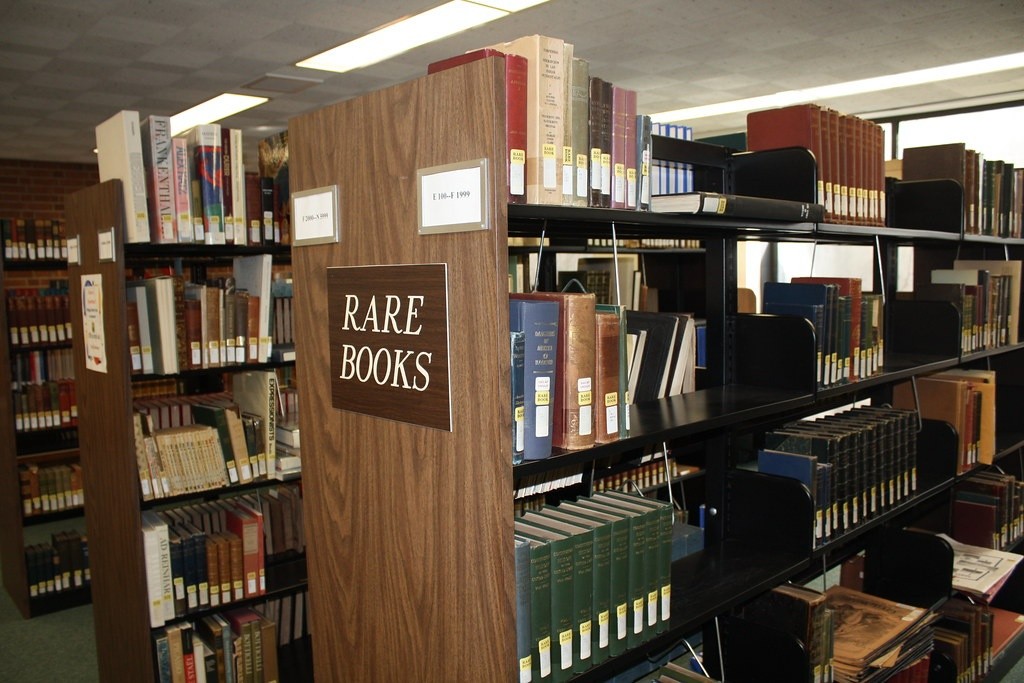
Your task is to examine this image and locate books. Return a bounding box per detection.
[509,258,1024,683]
[9,349,79,433]
[24,530,92,602]
[3,216,69,261]
[5,287,72,346]
[125,254,314,683]
[428,34,1023,238]
[95,110,292,246]
[19,462,85,518]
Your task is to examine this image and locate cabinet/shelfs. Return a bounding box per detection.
[4,52,1024,683]
